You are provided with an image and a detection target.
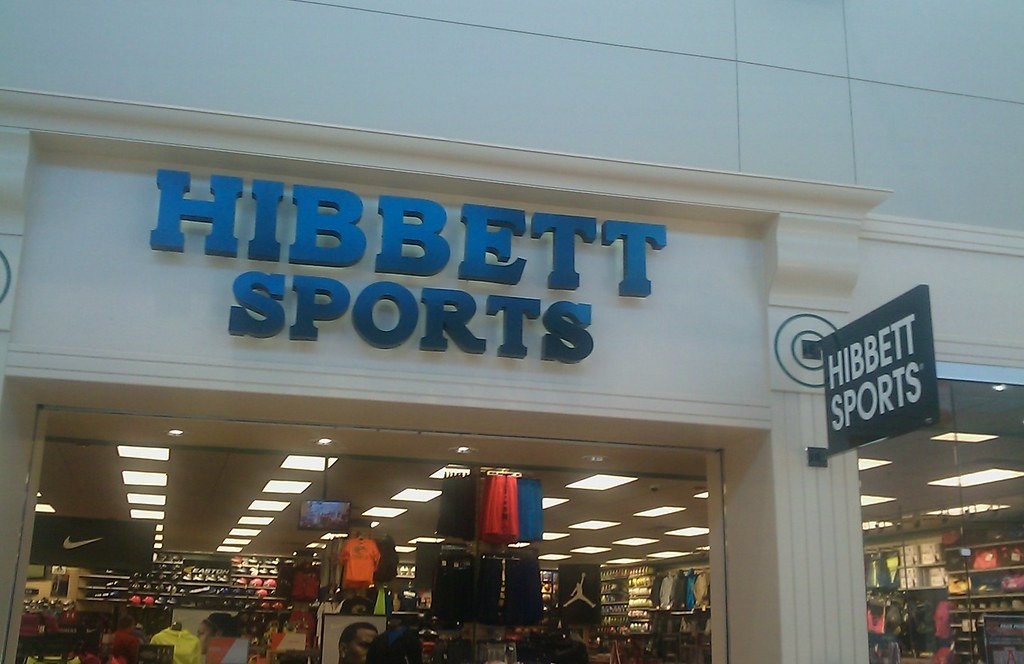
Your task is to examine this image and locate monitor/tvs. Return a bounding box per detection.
[298,500,351,533]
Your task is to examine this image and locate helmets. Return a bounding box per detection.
[23,597,74,611]
[110,554,293,613]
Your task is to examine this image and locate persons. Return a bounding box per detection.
[151,621,203,664]
[365,619,425,664]
[30,607,60,662]
[339,622,378,664]
[15,605,41,664]
[198,612,241,654]
[78,620,100,655]
[113,615,140,664]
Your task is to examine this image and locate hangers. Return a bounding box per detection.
[443,467,523,478]
[356,532,365,540]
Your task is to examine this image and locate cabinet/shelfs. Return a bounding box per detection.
[863,538,1024,664]
[594,564,713,664]
[76,554,558,664]
